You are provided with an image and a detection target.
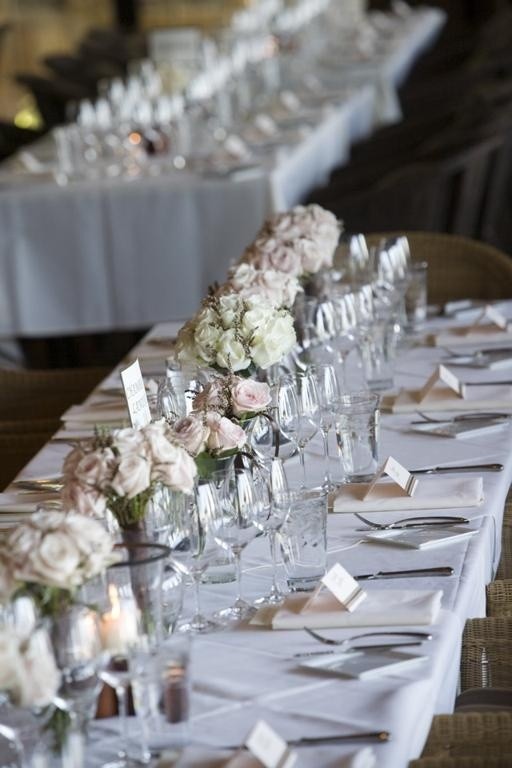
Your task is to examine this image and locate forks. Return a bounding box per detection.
[351,508,469,532]
[413,408,509,422]
[294,627,430,658]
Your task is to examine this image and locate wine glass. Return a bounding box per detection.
[259,232,412,492]
[48,3,368,189]
[0,466,328,767]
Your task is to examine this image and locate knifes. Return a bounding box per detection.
[194,728,395,752]
[340,568,459,580]
[380,461,504,477]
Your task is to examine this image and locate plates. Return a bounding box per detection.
[301,650,424,679]
[414,419,507,439]
[364,525,479,550]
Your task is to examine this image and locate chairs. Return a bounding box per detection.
[0,20,147,164]
